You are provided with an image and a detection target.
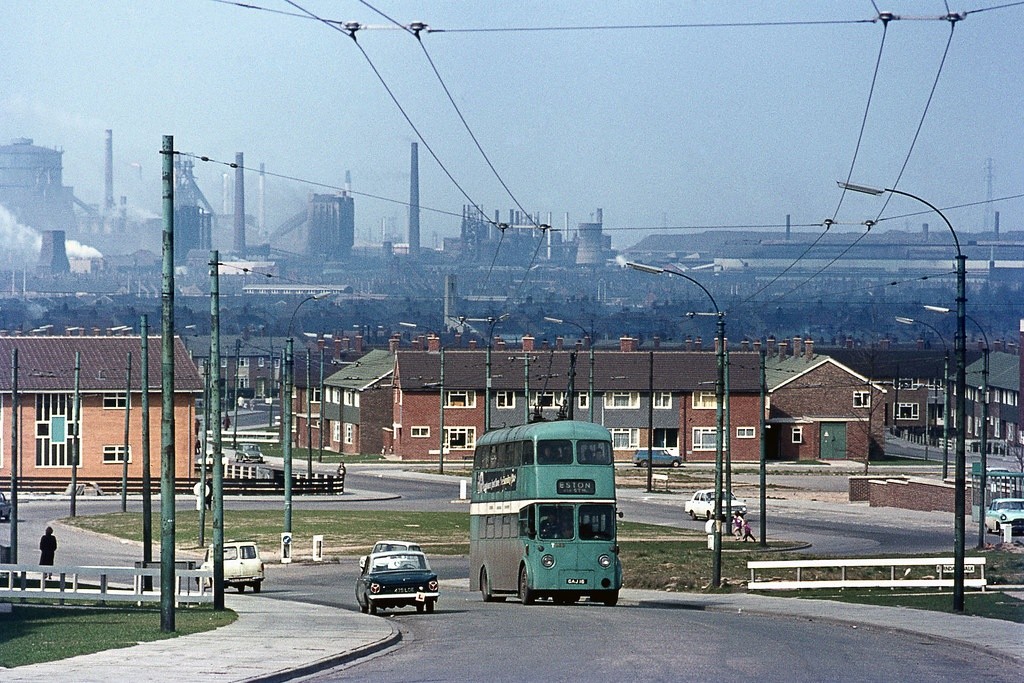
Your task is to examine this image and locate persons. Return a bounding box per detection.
[39,527,57,579]
[196,419,200,436]
[731,510,756,542]
[205,479,212,511]
[337,462,346,478]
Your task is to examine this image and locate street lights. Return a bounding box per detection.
[626,262,724,589]
[836,180,968,613]
[399,322,444,473]
[544,317,594,422]
[924,305,986,547]
[285,291,331,532]
[894,317,948,481]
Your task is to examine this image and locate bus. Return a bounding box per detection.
[470,422,623,606]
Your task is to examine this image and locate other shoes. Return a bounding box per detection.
[743,541,746,542]
[45,576,52,579]
[754,539,756,542]
[734,534,742,540]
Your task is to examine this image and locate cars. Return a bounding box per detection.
[684,489,747,521]
[632,449,682,467]
[985,498,1024,533]
[236,443,265,463]
[0,494,14,520]
[197,540,265,592]
[370,541,422,568]
[987,467,1016,483]
[355,551,440,615]
